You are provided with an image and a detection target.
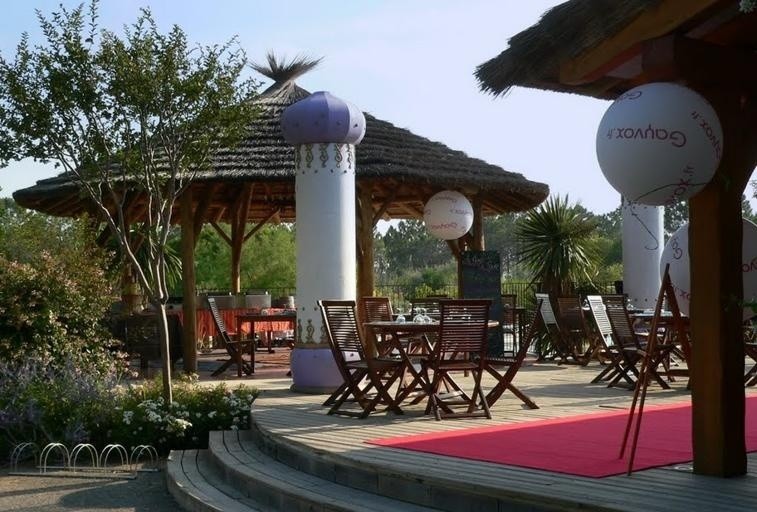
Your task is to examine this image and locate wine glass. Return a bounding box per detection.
[394,307,432,324]
[583,299,635,309]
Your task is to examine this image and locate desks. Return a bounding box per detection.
[362,318,501,420]
[235,313,297,376]
[166,308,296,353]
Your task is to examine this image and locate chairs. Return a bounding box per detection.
[356,294,425,403]
[410,298,434,318]
[317,298,407,418]
[532,293,757,394]
[420,299,494,419]
[476,298,542,411]
[495,295,519,357]
[207,298,259,377]
[427,294,448,312]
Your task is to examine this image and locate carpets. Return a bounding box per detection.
[363,395,757,478]
